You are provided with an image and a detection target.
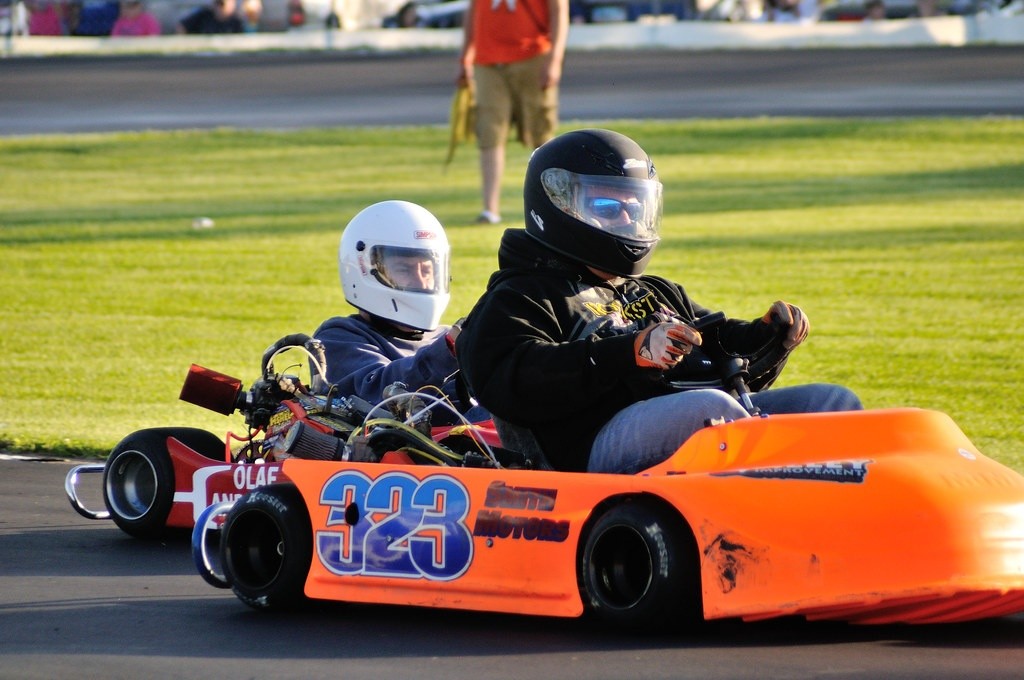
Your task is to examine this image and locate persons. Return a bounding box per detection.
[456,0,570,222]
[908,0,946,19]
[454,130,864,477]
[178,0,240,36]
[26,0,163,37]
[309,200,466,432]
[864,1,885,21]
[755,0,797,23]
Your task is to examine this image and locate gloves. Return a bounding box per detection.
[446,317,467,359]
[760,300,810,352]
[634,311,703,372]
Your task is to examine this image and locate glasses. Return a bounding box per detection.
[584,196,645,220]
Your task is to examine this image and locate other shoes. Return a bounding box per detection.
[478,211,502,225]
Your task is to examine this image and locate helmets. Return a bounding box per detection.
[523,128,664,279]
[339,200,451,332]
